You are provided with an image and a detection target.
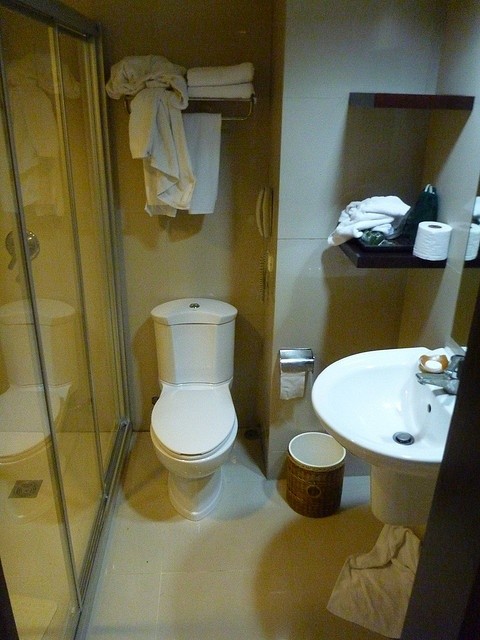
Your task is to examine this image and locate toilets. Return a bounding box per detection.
[150,297,239,521]
[0,297,85,524]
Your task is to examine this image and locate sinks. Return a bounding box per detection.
[311,346,471,534]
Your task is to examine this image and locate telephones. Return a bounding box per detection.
[253,188,272,239]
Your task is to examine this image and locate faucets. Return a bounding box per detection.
[417,355,464,395]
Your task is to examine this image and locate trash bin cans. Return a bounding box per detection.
[289,431,347,518]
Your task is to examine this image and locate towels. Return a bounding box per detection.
[0,48,82,217]
[186,61,256,87]
[187,82,256,99]
[105,53,196,218]
[325,522,423,639]
[327,194,412,247]
[182,112,223,215]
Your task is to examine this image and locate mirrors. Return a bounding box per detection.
[449,187,480,348]
